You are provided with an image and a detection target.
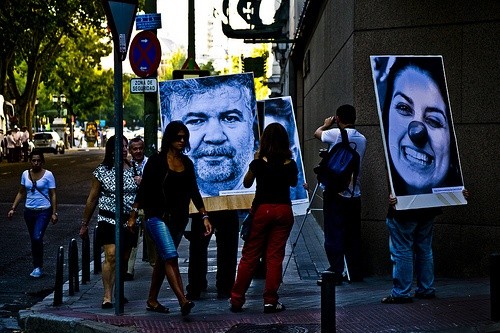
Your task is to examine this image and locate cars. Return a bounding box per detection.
[69,125,163,149]
[34,132,65,155]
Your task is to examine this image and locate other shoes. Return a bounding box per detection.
[186,289,231,301]
[230,301,285,314]
[30,267,45,277]
[382,292,435,303]
[102,293,128,308]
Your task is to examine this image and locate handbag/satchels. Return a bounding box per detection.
[240,212,252,240]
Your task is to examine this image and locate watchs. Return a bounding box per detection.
[53,212,59,216]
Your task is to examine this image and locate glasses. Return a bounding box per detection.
[176,134,187,142]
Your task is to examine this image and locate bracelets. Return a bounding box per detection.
[202,213,210,219]
[11,208,16,212]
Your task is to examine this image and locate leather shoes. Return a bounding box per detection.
[317,275,362,286]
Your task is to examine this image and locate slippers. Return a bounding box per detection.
[149,300,195,316]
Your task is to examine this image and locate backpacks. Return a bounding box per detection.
[313,127,360,198]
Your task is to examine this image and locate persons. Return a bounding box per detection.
[255,98,306,205]
[7,148,59,278]
[371,56,465,199]
[378,147,438,304]
[77,133,145,310]
[121,136,154,282]
[159,72,260,198]
[126,120,213,315]
[314,103,372,286]
[181,203,241,301]
[0,124,33,163]
[227,122,299,313]
